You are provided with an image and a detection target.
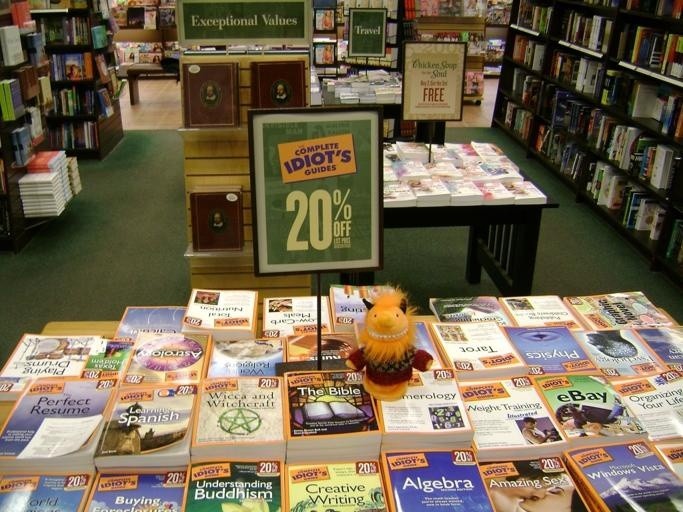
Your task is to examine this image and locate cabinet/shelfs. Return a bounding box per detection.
[487,0,680,285]
[180,52,311,298]
[399,1,486,106]
[1,1,126,254]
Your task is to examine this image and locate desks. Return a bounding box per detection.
[126,63,180,107]
[2,312,680,510]
[311,86,400,137]
[331,139,560,297]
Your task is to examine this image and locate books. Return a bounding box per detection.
[1,279,683,512]
[503,1,679,260]
[251,60,306,109]
[122,2,179,69]
[381,141,546,206]
[324,69,404,104]
[189,185,245,252]
[182,63,241,129]
[0,1,121,220]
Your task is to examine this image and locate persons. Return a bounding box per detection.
[273,85,289,102]
[211,211,226,231]
[205,86,217,104]
